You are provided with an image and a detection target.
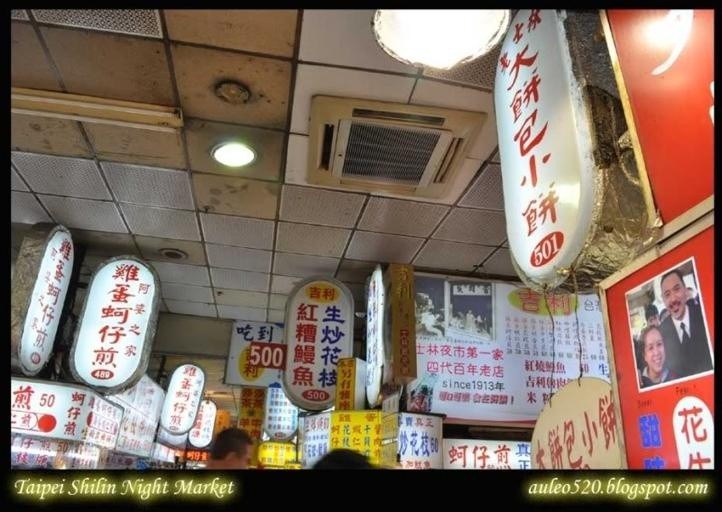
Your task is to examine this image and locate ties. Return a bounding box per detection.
[678,322,691,343]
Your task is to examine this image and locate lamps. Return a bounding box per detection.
[12,83,187,134]
[372,10,511,73]
[209,140,259,170]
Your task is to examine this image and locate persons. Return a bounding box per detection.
[205,427,255,470]
[633,268,713,388]
[415,296,490,342]
[313,448,373,469]
